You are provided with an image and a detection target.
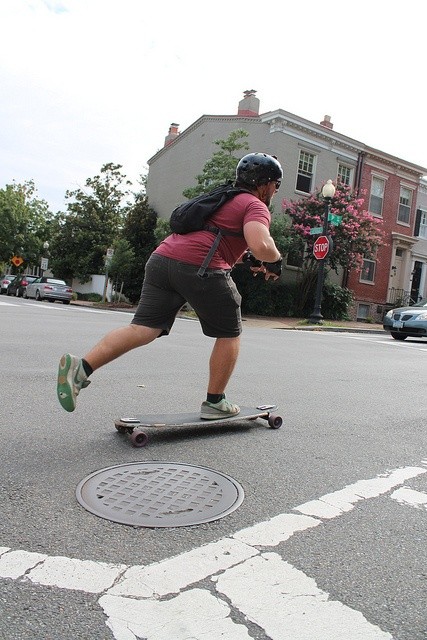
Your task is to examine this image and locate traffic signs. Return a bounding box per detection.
[327,213,342,227]
[309,227,322,235]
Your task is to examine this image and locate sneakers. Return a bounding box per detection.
[199,392,240,419]
[56,354,91,414]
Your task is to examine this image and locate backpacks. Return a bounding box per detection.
[169,179,259,276]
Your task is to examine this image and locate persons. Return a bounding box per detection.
[56,151,282,420]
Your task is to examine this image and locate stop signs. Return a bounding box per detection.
[312,236,329,260]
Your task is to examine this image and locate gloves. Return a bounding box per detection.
[261,254,282,277]
[246,251,261,268]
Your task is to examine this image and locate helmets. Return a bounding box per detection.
[235,152,282,187]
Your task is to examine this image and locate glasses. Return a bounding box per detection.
[271,180,280,189]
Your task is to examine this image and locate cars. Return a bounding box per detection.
[383,297,427,341]
[6,274,39,297]
[21,276,72,304]
[0,275,16,295]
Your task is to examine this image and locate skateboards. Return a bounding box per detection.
[116,404,282,446]
